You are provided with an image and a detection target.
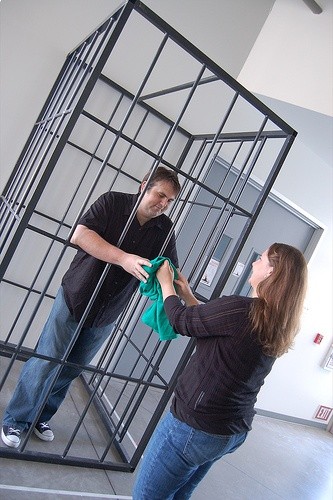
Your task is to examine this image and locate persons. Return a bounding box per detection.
[130,242,309,500]
[0,164,186,450]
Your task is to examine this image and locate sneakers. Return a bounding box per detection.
[1,424,23,449]
[30,421,55,442]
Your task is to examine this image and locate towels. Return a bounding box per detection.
[138,254,179,341]
